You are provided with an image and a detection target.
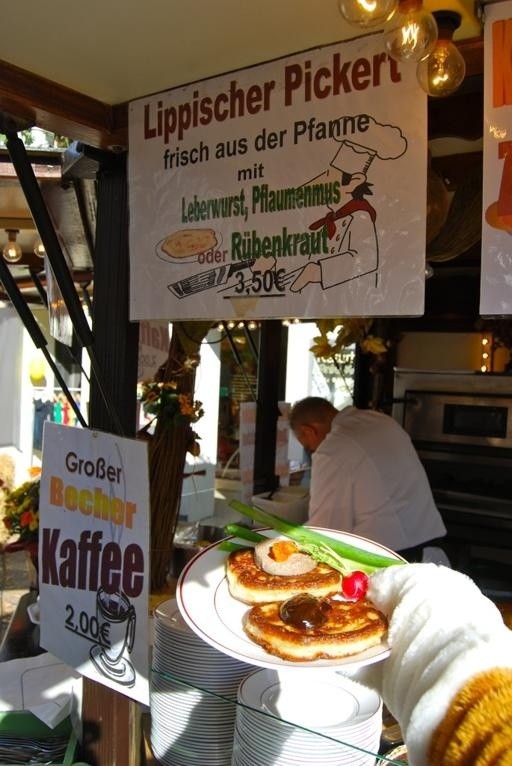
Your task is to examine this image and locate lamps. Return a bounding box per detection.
[33,237,46,258]
[415,9,467,98]
[1,229,23,263]
[339,0,399,30]
[384,0,439,66]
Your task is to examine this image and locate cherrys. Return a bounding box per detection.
[341,571,370,602]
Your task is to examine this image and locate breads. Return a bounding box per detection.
[227,535,345,604]
[241,592,390,665]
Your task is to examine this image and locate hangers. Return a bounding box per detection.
[53,391,80,404]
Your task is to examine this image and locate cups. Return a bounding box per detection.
[95,587,137,662]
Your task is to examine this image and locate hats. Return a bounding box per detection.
[330,114,408,175]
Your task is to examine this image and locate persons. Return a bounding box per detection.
[286,395,449,565]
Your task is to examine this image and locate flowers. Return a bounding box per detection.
[1,476,39,545]
[143,368,204,423]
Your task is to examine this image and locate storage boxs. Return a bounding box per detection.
[178,452,215,523]
[250,490,310,529]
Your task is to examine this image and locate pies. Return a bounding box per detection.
[161,228,218,259]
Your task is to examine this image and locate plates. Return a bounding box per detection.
[150,597,256,766]
[231,668,383,766]
[89,645,135,688]
[176,526,410,672]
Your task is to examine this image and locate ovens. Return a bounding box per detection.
[392,367,512,530]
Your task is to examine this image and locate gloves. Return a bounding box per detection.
[343,562,512,766]
